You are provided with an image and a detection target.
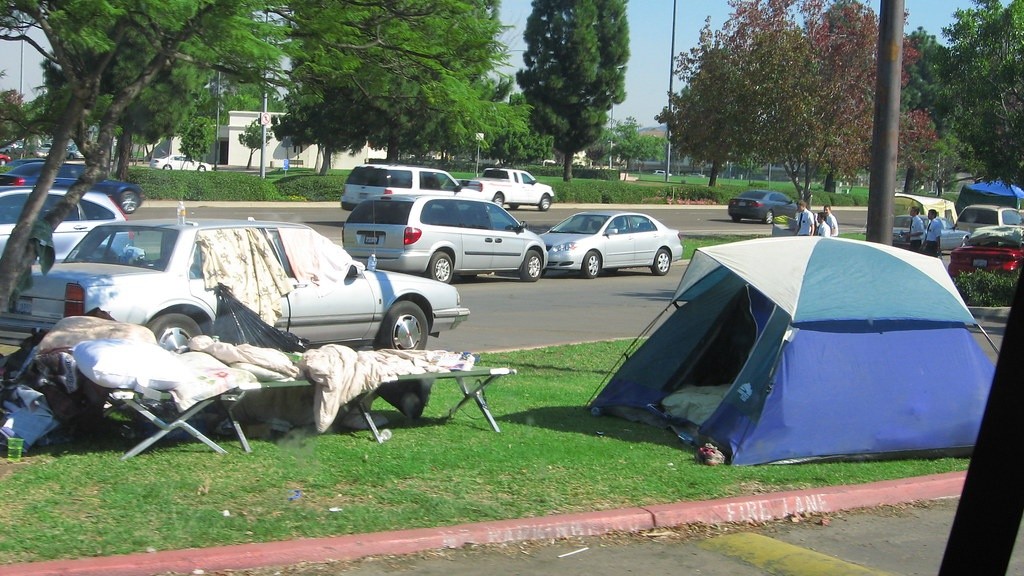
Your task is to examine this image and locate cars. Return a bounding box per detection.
[341,194,548,283]
[0,185,136,268]
[151,154,212,172]
[652,170,673,177]
[892,214,973,250]
[0,154,12,165]
[0,217,471,351]
[0,138,86,160]
[541,211,683,280]
[955,204,1024,234]
[0,161,146,215]
[948,225,1024,272]
[728,190,799,224]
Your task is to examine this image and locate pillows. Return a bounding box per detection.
[171,353,255,410]
[69,335,177,392]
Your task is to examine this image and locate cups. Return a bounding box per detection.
[7,438,24,462]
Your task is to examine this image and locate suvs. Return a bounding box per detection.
[339,162,491,212]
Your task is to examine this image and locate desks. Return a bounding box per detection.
[79,352,518,457]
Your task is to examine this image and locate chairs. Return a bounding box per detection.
[591,221,601,228]
[614,218,626,229]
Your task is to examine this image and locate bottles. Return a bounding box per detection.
[367,253,378,272]
[176,201,185,225]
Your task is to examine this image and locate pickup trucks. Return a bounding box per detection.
[452,167,555,212]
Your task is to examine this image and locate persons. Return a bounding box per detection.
[796,199,839,237]
[901,206,943,258]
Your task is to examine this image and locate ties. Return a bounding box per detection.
[910,219,912,232]
[799,213,803,230]
[925,222,931,240]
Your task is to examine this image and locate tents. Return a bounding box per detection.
[584,236,1001,467]
[894,180,1024,228]
